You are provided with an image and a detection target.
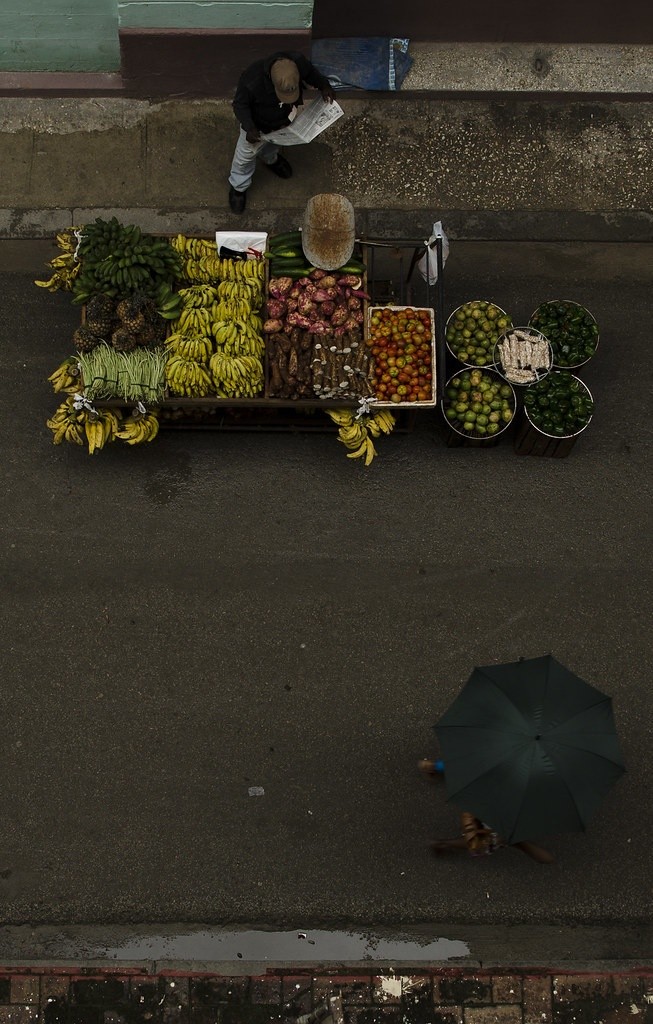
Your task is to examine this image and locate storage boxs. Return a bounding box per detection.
[444,300,599,458]
[368,306,436,410]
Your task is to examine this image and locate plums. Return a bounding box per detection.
[444,300,514,435]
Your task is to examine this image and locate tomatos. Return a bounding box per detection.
[365,307,433,403]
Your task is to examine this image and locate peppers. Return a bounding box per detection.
[523,300,600,436]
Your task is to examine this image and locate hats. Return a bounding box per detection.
[272,59,299,104]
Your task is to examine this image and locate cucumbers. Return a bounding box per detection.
[263,229,367,277]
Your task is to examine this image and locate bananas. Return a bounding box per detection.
[323,409,397,466]
[34,216,266,456]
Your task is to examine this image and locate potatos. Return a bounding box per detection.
[262,268,372,340]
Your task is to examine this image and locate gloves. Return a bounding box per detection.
[320,81,337,105]
[246,128,260,143]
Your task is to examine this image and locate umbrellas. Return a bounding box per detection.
[432,653,629,848]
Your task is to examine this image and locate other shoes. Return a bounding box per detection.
[264,153,293,179]
[229,185,247,214]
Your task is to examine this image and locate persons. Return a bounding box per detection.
[228,49,336,214]
[421,758,560,864]
